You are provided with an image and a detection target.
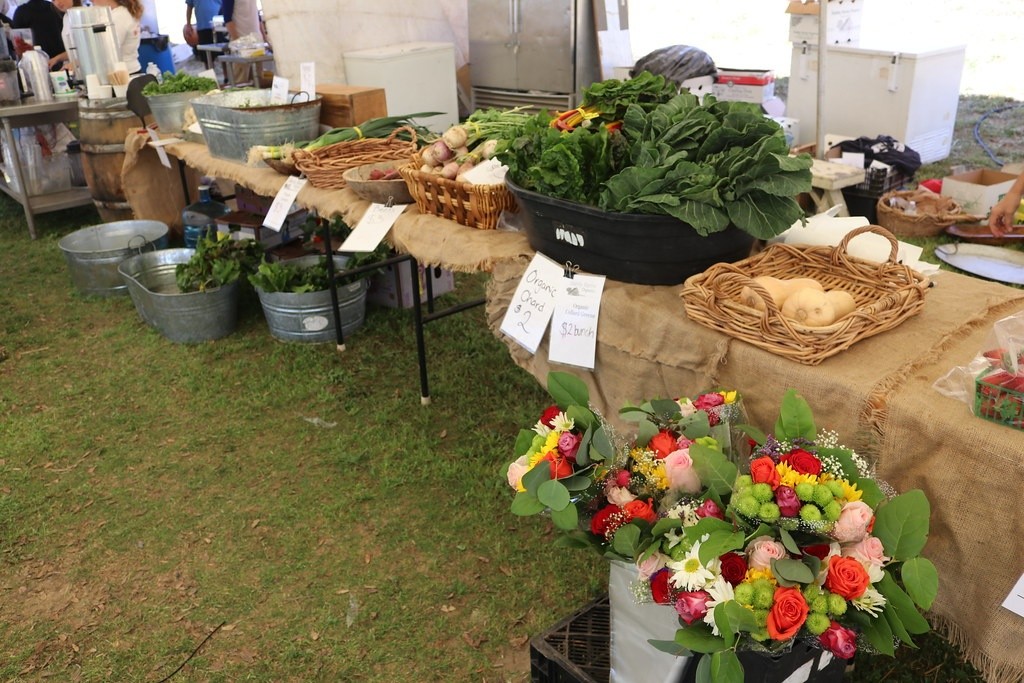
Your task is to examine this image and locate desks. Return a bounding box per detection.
[119,141,1024,683]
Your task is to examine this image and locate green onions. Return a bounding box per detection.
[246,112,447,164]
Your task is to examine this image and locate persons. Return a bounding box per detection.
[185,0,222,64]
[12,0,93,72]
[222,0,268,82]
[49,0,143,75]
[988,172,1024,238]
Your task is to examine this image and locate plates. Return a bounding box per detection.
[935,243,1024,285]
[945,224,1024,244]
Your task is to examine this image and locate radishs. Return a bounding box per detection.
[418,126,499,184]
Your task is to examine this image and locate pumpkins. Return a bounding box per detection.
[740,276,824,315]
[779,287,855,327]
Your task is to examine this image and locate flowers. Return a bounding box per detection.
[499,398,938,683]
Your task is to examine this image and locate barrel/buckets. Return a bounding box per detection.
[139,34,176,76]
[78,96,158,223]
[68,5,122,88]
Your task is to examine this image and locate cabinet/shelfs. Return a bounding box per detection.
[0,102,97,240]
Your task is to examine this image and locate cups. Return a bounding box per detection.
[23,51,71,101]
[85,75,129,99]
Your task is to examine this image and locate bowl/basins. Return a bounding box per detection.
[189,89,324,165]
[265,158,301,176]
[342,158,416,205]
[117,239,239,344]
[148,90,200,132]
[254,255,372,341]
[503,169,754,286]
[58,219,169,298]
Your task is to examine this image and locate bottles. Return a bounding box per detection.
[905,200,918,215]
[34,45,50,59]
[182,184,231,247]
[146,62,163,85]
[67,139,87,187]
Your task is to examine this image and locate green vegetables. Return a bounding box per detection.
[488,77,815,241]
[140,71,218,95]
[173,218,391,293]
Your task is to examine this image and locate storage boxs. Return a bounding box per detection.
[940,169,1020,227]
[505,169,755,287]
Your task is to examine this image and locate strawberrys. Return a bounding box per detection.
[977,347,1024,430]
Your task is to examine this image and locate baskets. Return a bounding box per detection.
[290,124,422,190]
[399,153,521,230]
[877,189,962,235]
[679,223,932,365]
[973,365,1024,431]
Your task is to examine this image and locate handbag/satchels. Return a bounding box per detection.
[829,136,922,178]
